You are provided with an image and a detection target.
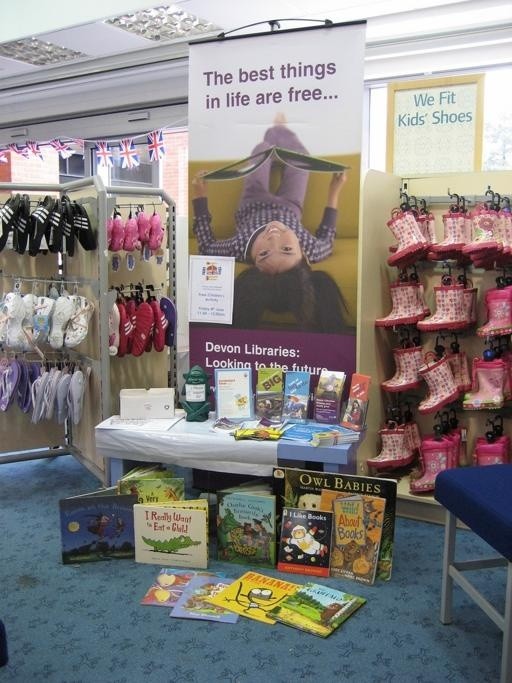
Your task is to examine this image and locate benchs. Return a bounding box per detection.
[93,406,369,488]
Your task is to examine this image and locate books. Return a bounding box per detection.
[315,369,346,422]
[60,487,138,564]
[279,506,334,579]
[319,489,386,585]
[278,419,359,446]
[140,569,226,608]
[205,576,305,624]
[216,485,279,569]
[214,369,255,423]
[170,577,242,624]
[118,465,187,501]
[210,419,283,441]
[341,374,370,431]
[266,582,368,639]
[271,467,397,583]
[134,499,210,568]
[284,371,310,424]
[256,368,284,419]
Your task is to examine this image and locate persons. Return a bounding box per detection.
[178,365,211,423]
[190,110,346,326]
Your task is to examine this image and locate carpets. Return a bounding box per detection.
[0,445,510,682]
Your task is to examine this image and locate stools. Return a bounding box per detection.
[432,460,512,682]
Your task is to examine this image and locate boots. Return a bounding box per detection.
[419,356,458,414]
[417,287,464,330]
[386,348,426,392]
[477,436,508,463]
[400,423,420,450]
[428,433,460,468]
[451,352,471,389]
[367,429,402,469]
[387,211,423,267]
[463,364,502,410]
[426,217,472,260]
[411,442,446,493]
[477,286,512,337]
[425,438,451,471]
[415,283,430,315]
[389,217,427,253]
[386,284,424,327]
[375,285,397,326]
[463,288,476,328]
[381,426,410,464]
[438,354,462,391]
[476,445,504,466]
[504,214,512,257]
[462,212,496,255]
[418,215,437,247]
[471,214,503,261]
[381,349,412,391]
[430,215,465,252]
[474,213,509,266]
[464,360,511,402]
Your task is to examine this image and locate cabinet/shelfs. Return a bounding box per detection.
[1,173,179,492]
[356,167,512,531]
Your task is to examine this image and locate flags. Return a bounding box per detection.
[95,141,113,167]
[27,141,43,160]
[146,131,165,161]
[7,145,27,158]
[51,140,74,159]
[0,149,10,165]
[118,140,140,168]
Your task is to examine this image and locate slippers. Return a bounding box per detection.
[22,364,40,414]
[17,195,30,254]
[47,367,58,383]
[0,361,21,411]
[49,199,61,252]
[12,194,19,251]
[73,205,95,252]
[131,304,155,356]
[30,196,53,255]
[160,298,175,343]
[61,195,71,213]
[151,301,168,353]
[62,201,73,256]
[124,218,139,250]
[31,371,47,425]
[138,213,150,240]
[17,359,29,408]
[111,216,125,252]
[57,374,71,426]
[0,193,20,255]
[126,301,135,341]
[108,304,119,356]
[106,218,113,249]
[67,372,84,425]
[117,304,132,355]
[149,214,163,248]
[44,370,61,421]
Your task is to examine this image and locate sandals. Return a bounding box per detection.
[49,298,72,346]
[64,296,94,348]
[32,298,54,342]
[0,292,16,342]
[21,293,36,346]
[6,296,27,346]
